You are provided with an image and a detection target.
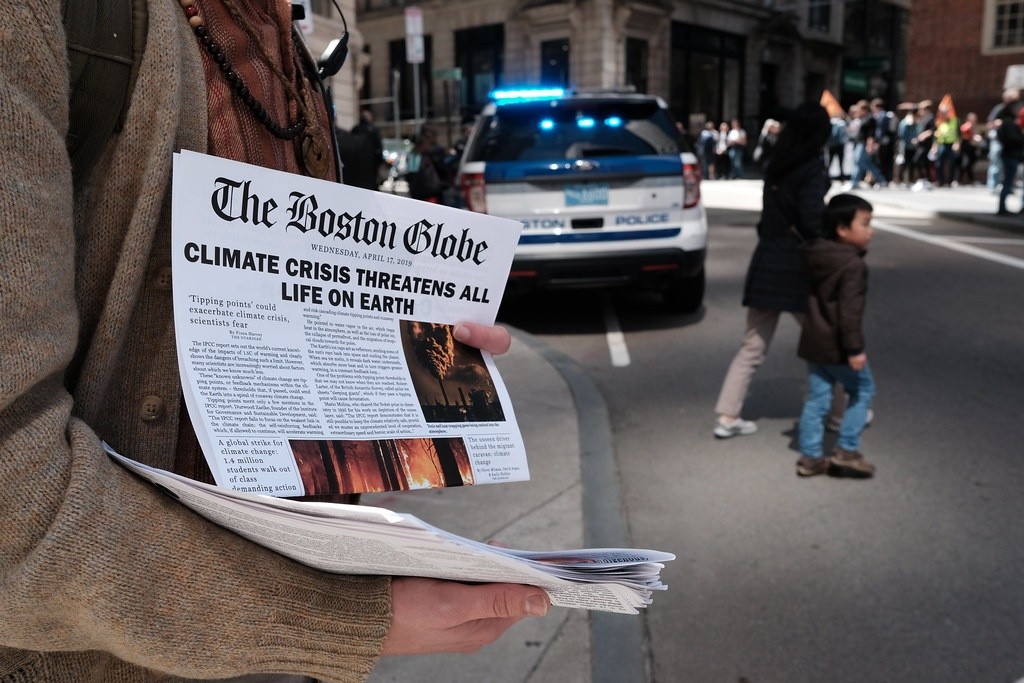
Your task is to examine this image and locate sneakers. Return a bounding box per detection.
[714,415,756,438]
[827,409,873,432]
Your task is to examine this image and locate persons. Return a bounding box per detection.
[711,103,873,437]
[349,109,382,188]
[677,86,1024,214]
[448,115,474,162]
[794,194,877,477]
[404,123,447,203]
[0,0,545,683]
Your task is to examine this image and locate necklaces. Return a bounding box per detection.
[181,0,330,178]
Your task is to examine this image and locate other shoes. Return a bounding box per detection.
[831,446,874,474]
[798,455,834,474]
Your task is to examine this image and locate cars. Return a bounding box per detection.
[441,83,711,318]
[373,136,420,202]
